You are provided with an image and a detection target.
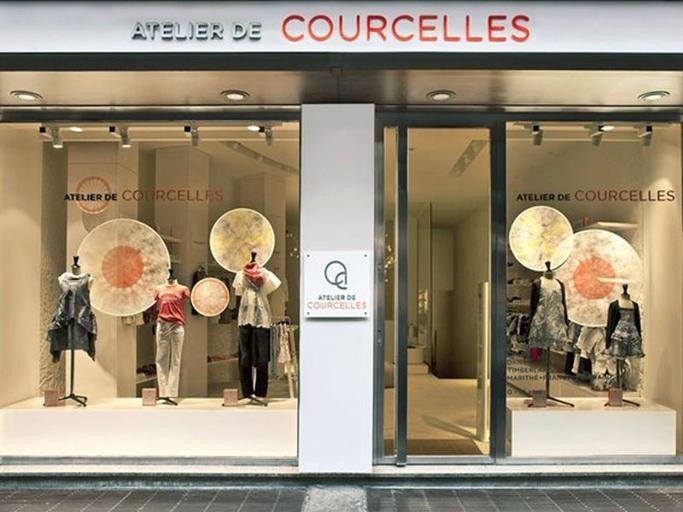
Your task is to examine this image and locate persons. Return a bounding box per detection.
[46,255,98,361]
[605,282,645,357]
[152,276,191,404]
[527,258,572,350]
[229,262,282,409]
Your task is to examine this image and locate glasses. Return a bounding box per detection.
[531,120,654,150]
[48,119,274,149]
